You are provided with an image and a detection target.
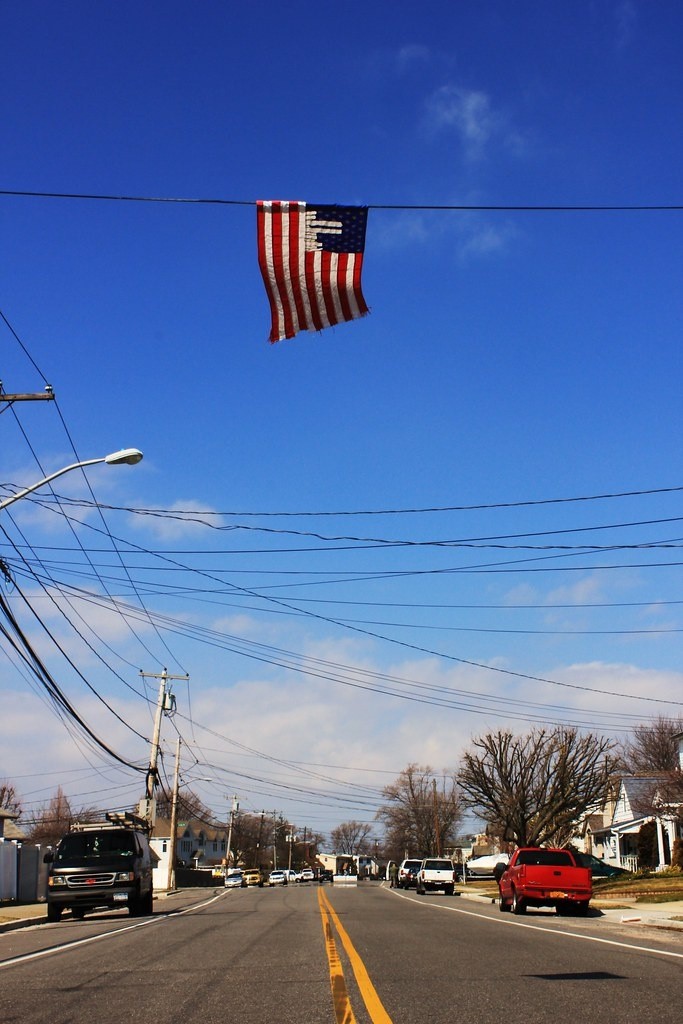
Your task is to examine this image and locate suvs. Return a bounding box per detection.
[319,870,333,883]
[243,869,264,887]
[386,858,423,886]
[416,858,456,896]
[268,870,287,886]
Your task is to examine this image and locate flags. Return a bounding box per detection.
[254,200,372,345]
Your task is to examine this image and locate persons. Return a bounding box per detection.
[389,864,399,888]
[340,862,357,876]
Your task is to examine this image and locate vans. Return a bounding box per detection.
[43,810,155,921]
[285,870,303,883]
[302,869,314,881]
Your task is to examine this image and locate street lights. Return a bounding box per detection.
[226,812,267,859]
[167,777,211,890]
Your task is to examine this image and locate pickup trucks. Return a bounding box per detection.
[497,845,593,915]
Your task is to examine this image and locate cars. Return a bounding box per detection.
[573,853,626,878]
[405,867,419,889]
[224,873,243,888]
